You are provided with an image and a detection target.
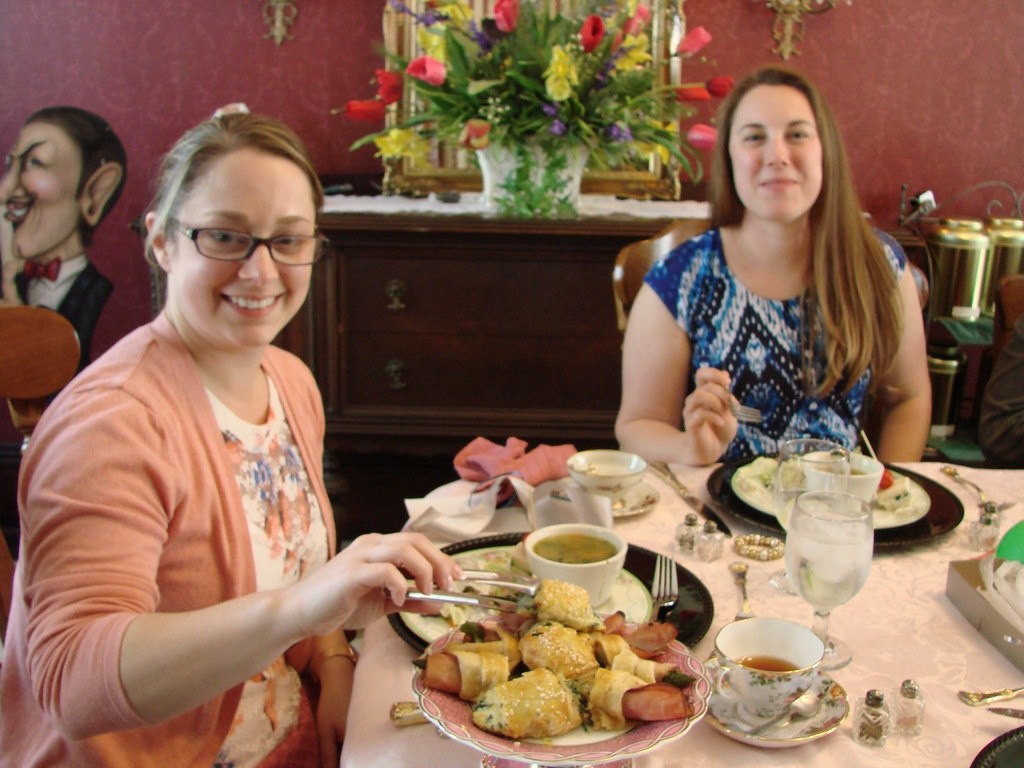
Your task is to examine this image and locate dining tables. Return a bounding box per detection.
[337,460,1024,768]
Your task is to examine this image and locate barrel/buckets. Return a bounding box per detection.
[928,217,1024,321]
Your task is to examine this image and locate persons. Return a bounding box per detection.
[0,103,463,768]
[976,273,1024,469]
[615,65,933,467]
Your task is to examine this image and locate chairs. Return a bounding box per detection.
[616,209,929,349]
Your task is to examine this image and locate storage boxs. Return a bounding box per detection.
[943,551,1024,675]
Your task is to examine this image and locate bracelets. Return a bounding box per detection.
[309,644,358,683]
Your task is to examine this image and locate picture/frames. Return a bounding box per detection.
[381,0,683,200]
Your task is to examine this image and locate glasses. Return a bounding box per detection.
[170,217,331,265]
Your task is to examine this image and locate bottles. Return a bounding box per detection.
[891,678,928,737]
[852,689,892,749]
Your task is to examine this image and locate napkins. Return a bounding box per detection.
[454,435,579,506]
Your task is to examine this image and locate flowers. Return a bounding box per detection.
[348,0,734,203]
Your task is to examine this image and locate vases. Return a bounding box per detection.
[473,145,587,212]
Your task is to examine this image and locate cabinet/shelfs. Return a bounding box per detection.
[306,173,709,514]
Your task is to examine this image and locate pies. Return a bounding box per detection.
[472,578,609,744]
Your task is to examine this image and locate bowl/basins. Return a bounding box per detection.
[565,448,648,501]
[801,450,885,501]
[524,523,628,607]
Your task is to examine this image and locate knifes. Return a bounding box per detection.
[648,461,734,540]
[986,708,1024,719]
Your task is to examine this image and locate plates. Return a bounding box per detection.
[386,531,714,654]
[701,656,851,748]
[399,546,653,642]
[731,456,932,531]
[556,477,661,518]
[410,611,713,768]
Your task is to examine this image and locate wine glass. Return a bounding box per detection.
[783,490,874,670]
[769,437,851,532]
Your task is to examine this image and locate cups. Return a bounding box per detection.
[713,616,825,717]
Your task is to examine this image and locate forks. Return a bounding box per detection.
[650,553,679,621]
